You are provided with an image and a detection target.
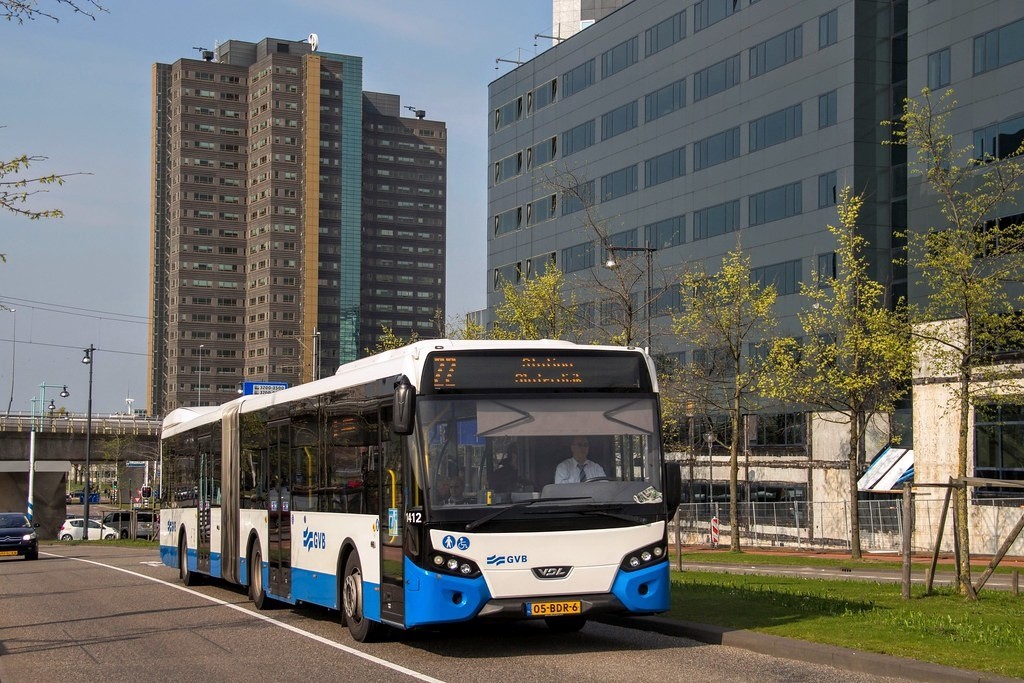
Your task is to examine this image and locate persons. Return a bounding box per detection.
[554,434,609,486]
[491,442,522,493]
[425,474,474,507]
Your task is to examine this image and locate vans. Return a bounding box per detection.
[101,511,159,540]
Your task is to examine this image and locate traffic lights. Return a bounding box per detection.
[147,487,152,498]
[142,486,147,497]
[113,481,117,489]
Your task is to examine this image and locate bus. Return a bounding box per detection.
[158,334,687,654]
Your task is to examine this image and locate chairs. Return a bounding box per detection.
[549,445,597,484]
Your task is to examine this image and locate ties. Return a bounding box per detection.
[577,463,587,483]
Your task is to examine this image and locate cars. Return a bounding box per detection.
[680,481,807,502]
[0,512,40,560]
[66,493,72,505]
[58,518,120,541]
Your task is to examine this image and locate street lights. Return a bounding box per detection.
[195,344,205,406]
[81,343,97,541]
[702,432,718,545]
[27,397,55,521]
[39,381,69,432]
[602,243,662,353]
